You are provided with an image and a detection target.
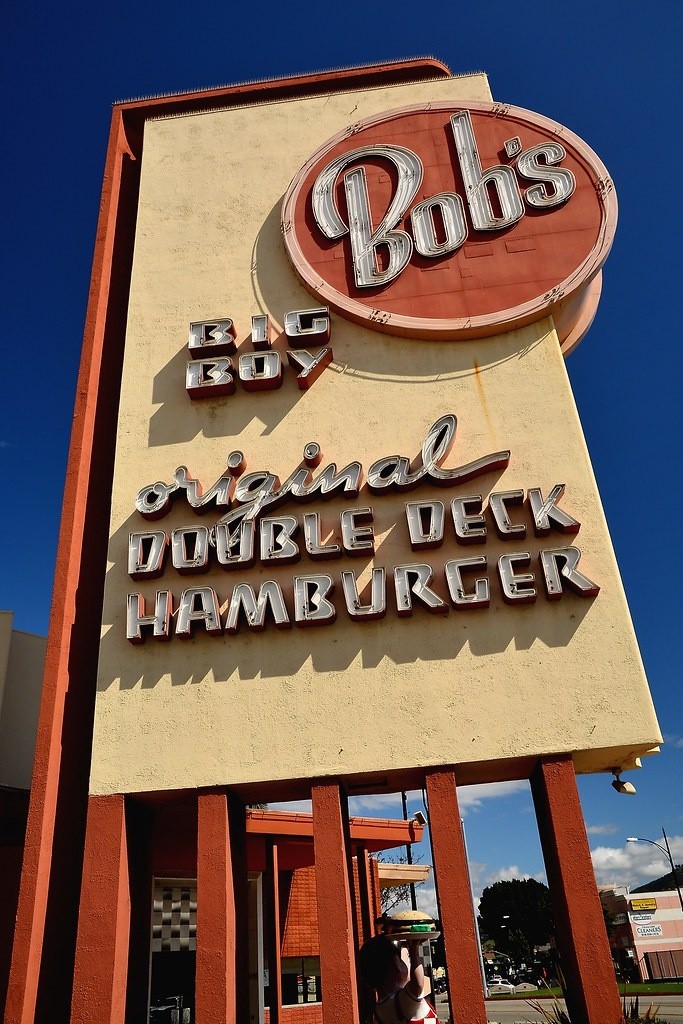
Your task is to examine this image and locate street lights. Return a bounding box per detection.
[626,826,683,911]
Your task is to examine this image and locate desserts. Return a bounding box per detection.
[376,910,436,932]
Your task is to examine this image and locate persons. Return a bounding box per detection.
[357,933,442,1024]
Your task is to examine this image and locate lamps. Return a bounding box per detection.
[609,766,637,795]
[408,811,427,825]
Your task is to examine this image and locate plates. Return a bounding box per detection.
[384,931,441,939]
